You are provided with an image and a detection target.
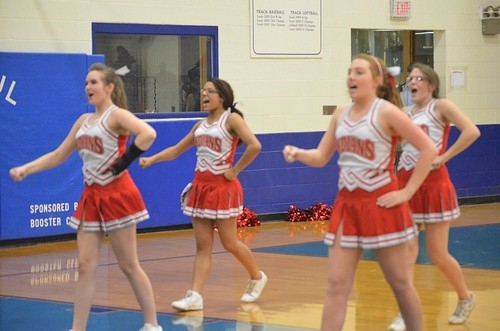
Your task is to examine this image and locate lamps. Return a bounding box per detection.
[480,5,500,35]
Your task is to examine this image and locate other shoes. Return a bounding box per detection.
[139,323,163,331]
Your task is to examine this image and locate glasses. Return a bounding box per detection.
[406,76,429,84]
[200,88,218,95]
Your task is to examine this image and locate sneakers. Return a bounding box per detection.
[171,290,203,310]
[448,291,476,324]
[389,313,407,331]
[242,303,265,330]
[241,271,267,302]
[172,311,204,328]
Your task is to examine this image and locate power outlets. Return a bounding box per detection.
[323,105,337,115]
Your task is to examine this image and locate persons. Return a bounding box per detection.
[9,66,169,331]
[139,78,269,310]
[282,54,440,331]
[388,62,480,331]
